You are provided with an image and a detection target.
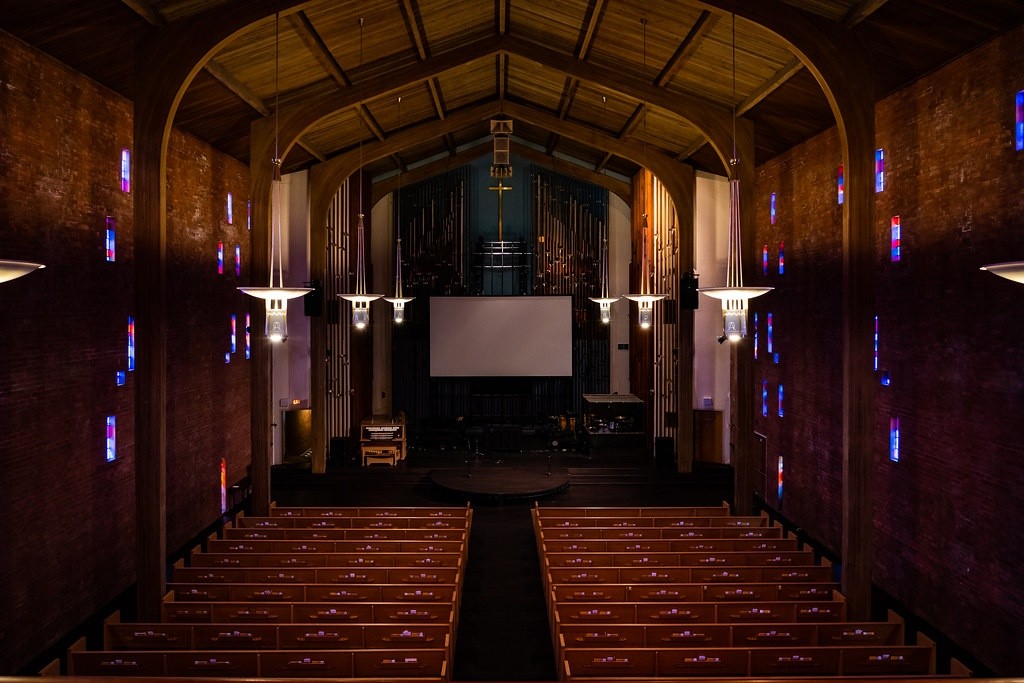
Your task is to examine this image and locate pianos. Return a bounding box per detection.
[358,410,406,460]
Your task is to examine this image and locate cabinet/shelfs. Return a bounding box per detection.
[581,394,647,459]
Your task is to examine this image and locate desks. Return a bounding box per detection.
[359,413,407,462]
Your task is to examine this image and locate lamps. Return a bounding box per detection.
[623,19,667,330]
[236,9,314,343]
[336,17,382,330]
[587,95,621,325]
[385,95,415,324]
[693,8,776,345]
[0,260,46,284]
[979,261,1024,284]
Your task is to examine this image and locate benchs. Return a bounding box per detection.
[361,445,398,468]
[531,502,974,681]
[39,502,471,678]
